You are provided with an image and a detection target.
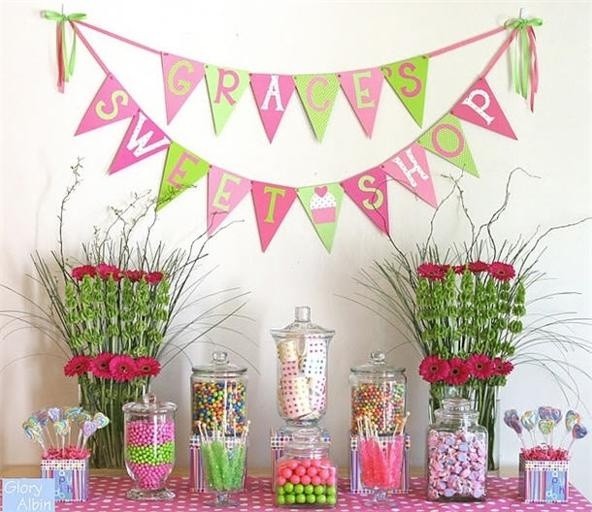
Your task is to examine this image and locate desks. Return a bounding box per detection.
[1,463,592,511]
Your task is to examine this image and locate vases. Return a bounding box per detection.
[73,368,154,481]
[425,380,503,477]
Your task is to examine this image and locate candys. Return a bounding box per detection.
[125,418,176,490]
[503,406,588,440]
[426,426,488,500]
[351,380,406,434]
[277,335,327,420]
[200,440,246,492]
[22,407,109,443]
[274,457,336,506]
[360,434,404,489]
[190,381,248,437]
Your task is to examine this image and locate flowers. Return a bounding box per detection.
[332,160,592,467]
[0,153,262,465]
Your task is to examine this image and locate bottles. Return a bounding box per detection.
[121,392,179,503]
[269,305,336,436]
[272,427,337,509]
[189,349,249,439]
[347,350,408,437]
[424,391,489,503]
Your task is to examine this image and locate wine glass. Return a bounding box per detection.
[196,437,250,509]
[355,437,407,511]
